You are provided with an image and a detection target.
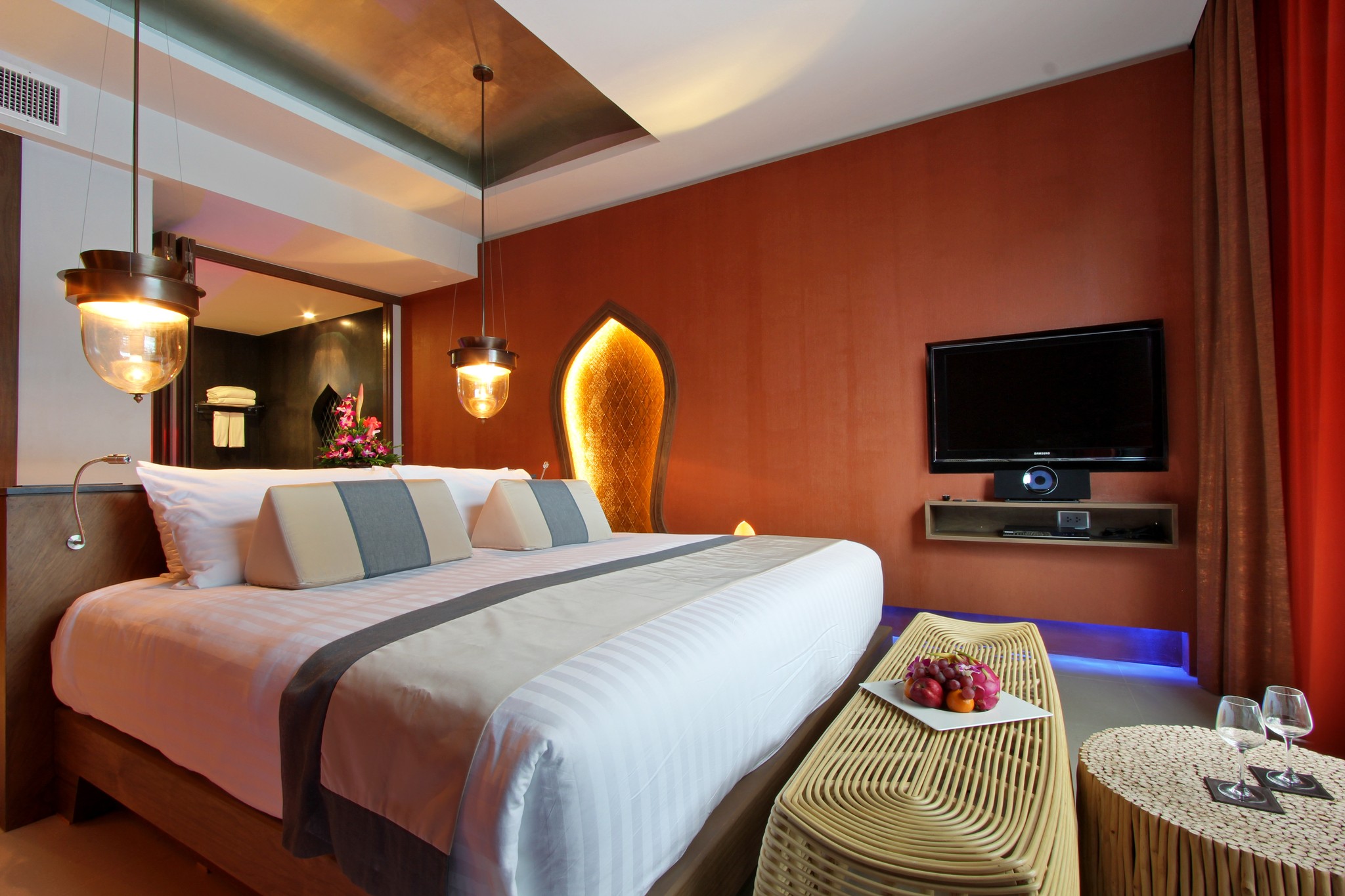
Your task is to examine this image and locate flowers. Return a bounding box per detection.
[310,382,405,471]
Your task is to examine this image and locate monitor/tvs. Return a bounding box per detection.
[924,318,1169,474]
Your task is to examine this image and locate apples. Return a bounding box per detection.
[909,677,944,708]
[894,677,915,699]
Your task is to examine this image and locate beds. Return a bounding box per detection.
[2,482,895,896]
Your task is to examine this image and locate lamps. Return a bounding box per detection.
[445,64,520,424]
[57,0,209,404]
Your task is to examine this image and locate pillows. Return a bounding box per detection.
[244,479,473,593]
[135,456,191,581]
[467,477,614,553]
[134,465,400,601]
[394,464,536,543]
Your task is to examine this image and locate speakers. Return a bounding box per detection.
[992,464,1091,504]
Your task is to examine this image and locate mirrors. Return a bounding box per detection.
[187,245,393,470]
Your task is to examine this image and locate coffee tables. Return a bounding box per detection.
[746,611,1086,896]
[1076,720,1345,896]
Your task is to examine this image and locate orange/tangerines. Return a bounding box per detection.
[946,688,974,713]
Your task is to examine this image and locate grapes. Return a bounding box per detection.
[912,659,974,699]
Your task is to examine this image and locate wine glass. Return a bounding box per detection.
[1216,684,1316,803]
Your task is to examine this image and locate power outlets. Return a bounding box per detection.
[1058,510,1090,529]
